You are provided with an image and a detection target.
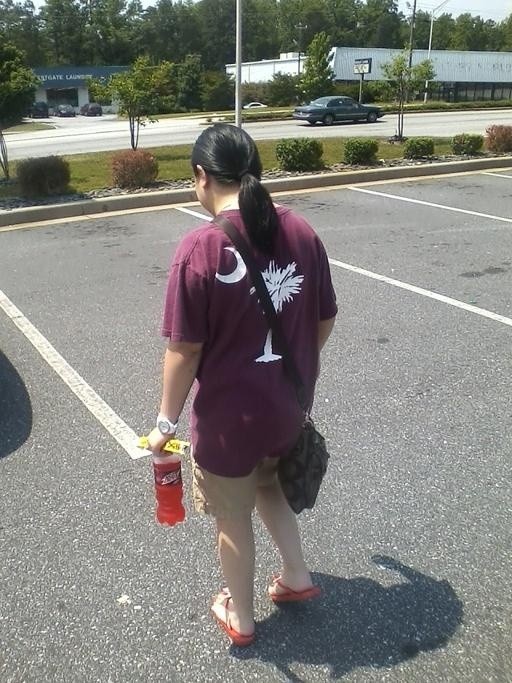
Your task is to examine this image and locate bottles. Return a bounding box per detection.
[151,447,186,527]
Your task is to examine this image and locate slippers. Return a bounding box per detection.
[209,591,256,647]
[267,575,320,603]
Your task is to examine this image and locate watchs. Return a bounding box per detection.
[156,413,179,435]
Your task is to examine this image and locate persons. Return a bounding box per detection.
[145,122,338,645]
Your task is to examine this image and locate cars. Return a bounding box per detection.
[242,101,268,109]
[291,95,387,126]
[29,100,102,119]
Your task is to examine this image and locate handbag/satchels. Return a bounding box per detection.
[274,415,331,515]
